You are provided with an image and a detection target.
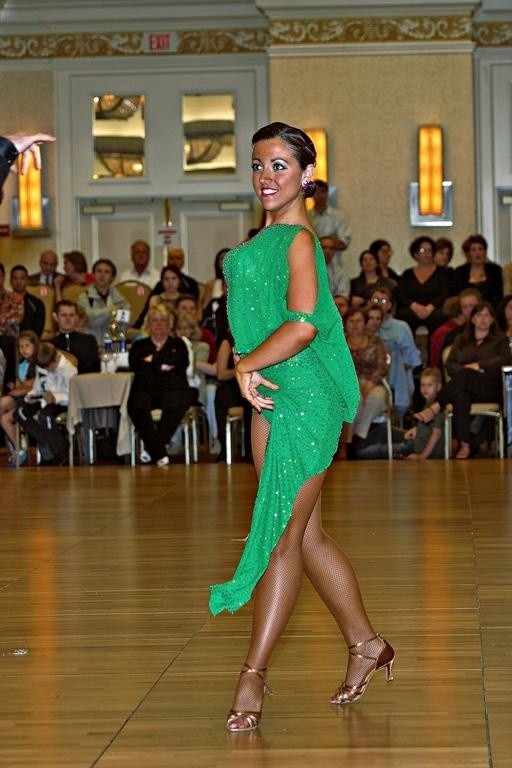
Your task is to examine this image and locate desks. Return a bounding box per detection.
[100,351,129,373]
[69,373,135,465]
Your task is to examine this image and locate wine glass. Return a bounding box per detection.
[101,348,113,376]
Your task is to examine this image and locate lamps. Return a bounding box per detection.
[12,141,51,235]
[409,123,454,226]
[305,126,338,210]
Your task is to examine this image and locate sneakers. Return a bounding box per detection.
[140,449,169,466]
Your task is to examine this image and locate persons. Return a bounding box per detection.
[206,122,394,732]
[0,131,58,212]
[1,180,511,465]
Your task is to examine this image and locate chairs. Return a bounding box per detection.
[13,347,81,465]
[129,335,200,465]
[222,403,243,465]
[438,343,506,463]
[372,344,397,460]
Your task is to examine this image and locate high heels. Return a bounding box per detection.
[329,633,395,705]
[224,661,273,732]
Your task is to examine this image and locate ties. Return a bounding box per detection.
[45,275,49,285]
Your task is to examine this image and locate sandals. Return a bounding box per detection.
[410,403,438,428]
